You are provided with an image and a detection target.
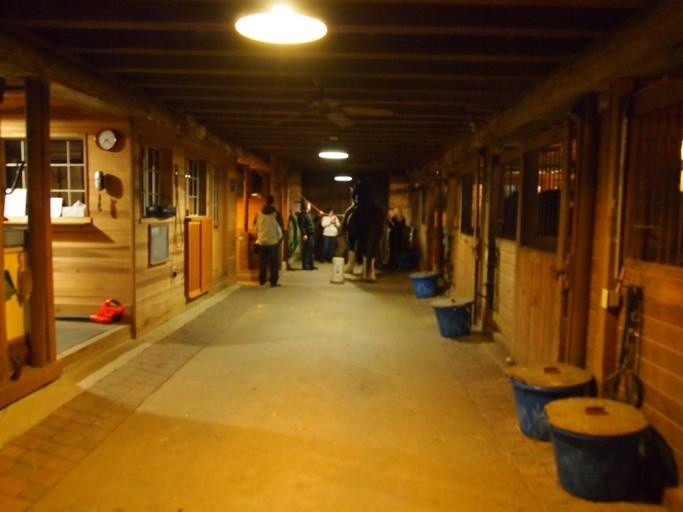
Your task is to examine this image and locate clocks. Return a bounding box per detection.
[96,128,118,150]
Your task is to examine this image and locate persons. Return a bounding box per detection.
[253,177,407,287]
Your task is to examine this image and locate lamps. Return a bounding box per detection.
[334,175,352,182]
[318,135,349,160]
[234,0,328,45]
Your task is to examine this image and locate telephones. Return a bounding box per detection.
[94,171,103,191]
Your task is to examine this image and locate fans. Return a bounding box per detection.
[264,77,396,129]
